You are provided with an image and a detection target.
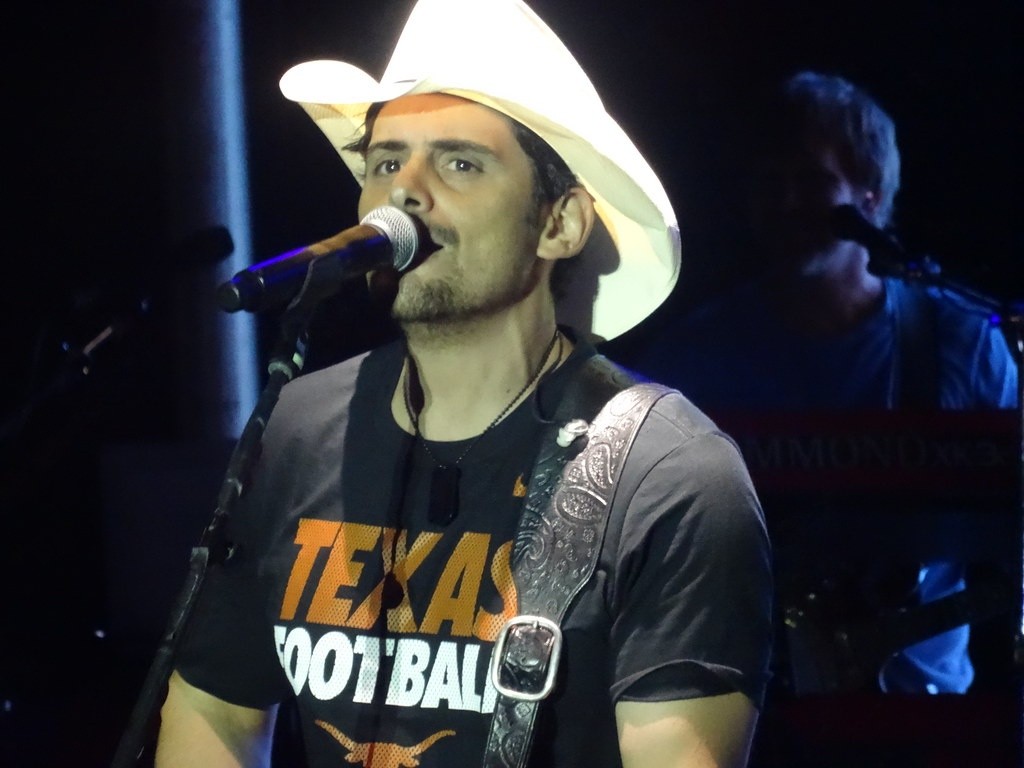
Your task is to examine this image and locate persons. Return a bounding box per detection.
[153,0,772,767]
[631,71,1024,768]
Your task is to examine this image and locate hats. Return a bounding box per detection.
[279,0,682,343]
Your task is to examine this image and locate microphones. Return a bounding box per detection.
[217,204,419,313]
[831,204,908,262]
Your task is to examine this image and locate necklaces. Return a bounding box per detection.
[403,327,558,527]
